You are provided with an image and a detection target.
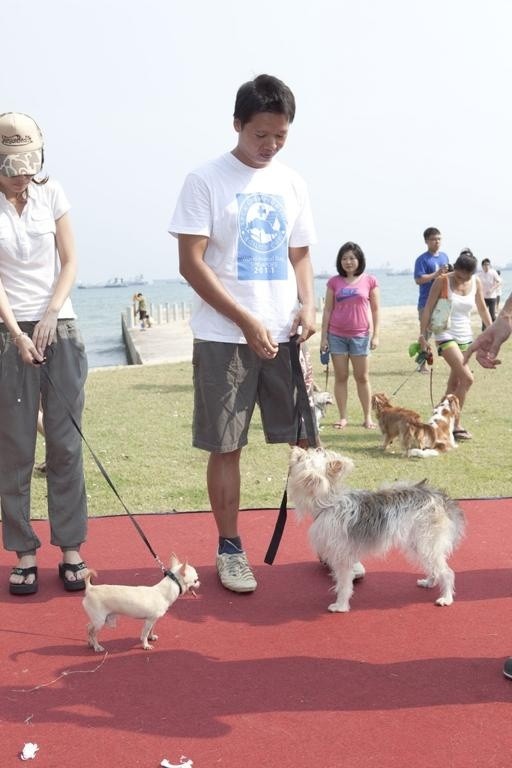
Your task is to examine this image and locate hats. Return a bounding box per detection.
[0,112,44,177]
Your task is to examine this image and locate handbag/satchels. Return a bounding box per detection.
[425,275,453,334]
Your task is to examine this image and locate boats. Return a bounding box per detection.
[386,268,413,276]
[314,270,333,279]
[77,273,191,289]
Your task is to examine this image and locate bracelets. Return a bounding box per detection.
[13,332,28,347]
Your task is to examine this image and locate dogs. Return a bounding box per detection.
[371,393,436,458]
[422,394,460,451]
[82,552,201,653]
[283,445,468,613]
[302,391,335,434]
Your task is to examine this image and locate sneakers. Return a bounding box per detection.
[215,543,257,594]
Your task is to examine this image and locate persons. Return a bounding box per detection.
[134,292,152,329]
[1,111,93,596]
[320,242,379,429]
[501,654,511,681]
[413,228,512,441]
[168,74,366,595]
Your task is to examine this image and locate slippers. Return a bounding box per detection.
[334,418,348,428]
[363,421,377,429]
[9,566,40,595]
[58,560,91,590]
[453,427,473,441]
